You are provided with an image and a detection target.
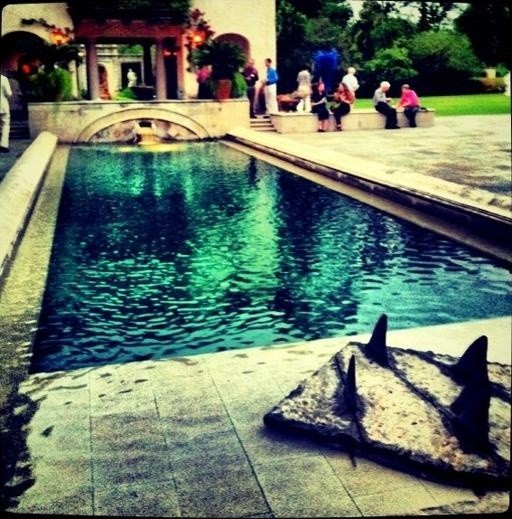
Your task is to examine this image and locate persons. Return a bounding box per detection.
[0,74,12,153]
[372,81,400,129]
[330,84,351,131]
[297,64,311,114]
[310,84,330,133]
[398,85,418,128]
[264,60,277,116]
[243,60,258,119]
[128,69,138,90]
[197,64,209,99]
[342,68,358,112]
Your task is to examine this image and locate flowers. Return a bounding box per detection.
[184,8,214,51]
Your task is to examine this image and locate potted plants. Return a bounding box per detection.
[192,39,248,99]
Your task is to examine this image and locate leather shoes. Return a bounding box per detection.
[251,116,257,118]
[263,116,270,118]
